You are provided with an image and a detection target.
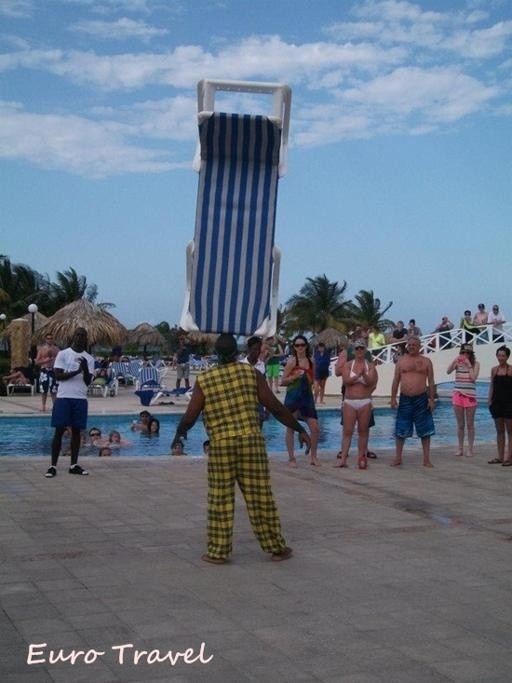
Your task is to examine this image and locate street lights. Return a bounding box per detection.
[1,313,8,358]
[28,303,39,358]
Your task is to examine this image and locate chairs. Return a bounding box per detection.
[6,353,218,406]
[177,79,293,338]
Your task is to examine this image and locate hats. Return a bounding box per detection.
[461,344,473,352]
[354,339,365,347]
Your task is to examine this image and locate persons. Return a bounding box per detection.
[170,332,313,565]
[389,334,436,468]
[280,335,321,469]
[45,326,95,478]
[330,338,379,469]
[445,342,481,459]
[2,300,507,457]
[485,344,511,468]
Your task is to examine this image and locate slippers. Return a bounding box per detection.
[367,451,376,457]
[488,458,503,463]
[502,460,512,465]
[337,452,349,457]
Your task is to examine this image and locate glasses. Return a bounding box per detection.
[295,344,305,346]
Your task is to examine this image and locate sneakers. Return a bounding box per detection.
[44,465,56,476]
[70,464,87,475]
